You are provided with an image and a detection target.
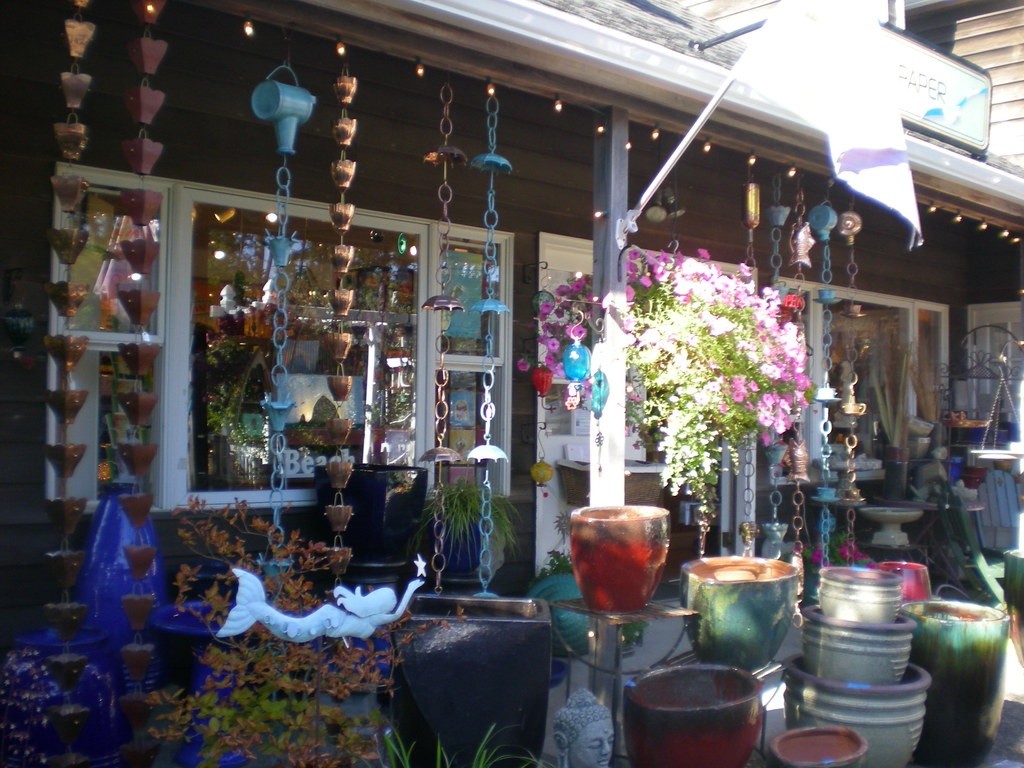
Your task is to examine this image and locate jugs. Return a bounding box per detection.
[808,201,837,241]
[251,65,317,155]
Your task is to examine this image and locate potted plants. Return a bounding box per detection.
[408,475,520,577]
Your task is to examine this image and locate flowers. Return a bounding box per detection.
[790,531,876,574]
[516,244,814,498]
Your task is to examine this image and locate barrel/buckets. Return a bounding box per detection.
[391,593,554,768]
[782,653,931,768]
[569,505,671,614]
[681,556,800,671]
[900,602,1010,768]
[768,725,868,768]
[622,665,762,768]
[818,568,904,622]
[797,606,918,683]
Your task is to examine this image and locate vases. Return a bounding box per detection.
[315,464,428,561]
[771,727,868,768]
[783,652,933,768]
[861,507,925,545]
[820,567,903,627]
[0,626,125,768]
[623,664,764,768]
[899,601,1010,768]
[803,605,916,684]
[680,556,798,670]
[393,617,550,768]
[73,490,168,696]
[151,601,280,768]
[569,505,671,615]
